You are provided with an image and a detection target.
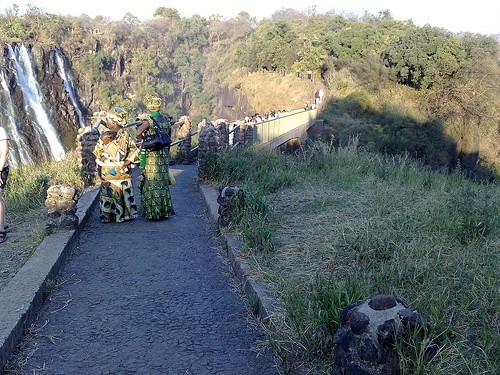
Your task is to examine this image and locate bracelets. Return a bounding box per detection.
[0,171,3,172]
[0,174,1,175]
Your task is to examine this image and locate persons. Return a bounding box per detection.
[92,106,141,223]
[100,130,125,176]
[0,126,10,243]
[135,95,177,221]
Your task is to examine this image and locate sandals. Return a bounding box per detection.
[4,225,10,233]
[0,230,7,242]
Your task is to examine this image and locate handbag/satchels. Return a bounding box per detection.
[141,116,172,152]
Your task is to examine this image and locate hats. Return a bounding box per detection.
[145,95,162,113]
[106,107,128,128]
